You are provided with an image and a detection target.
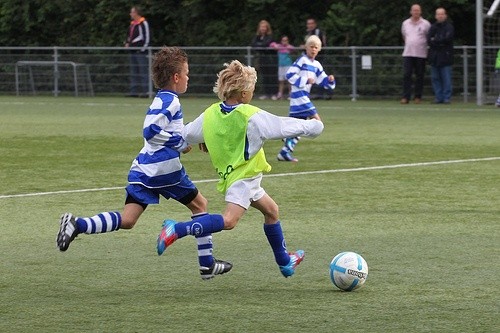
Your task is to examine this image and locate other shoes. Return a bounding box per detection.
[496,98,500,106]
[414,97,421,104]
[435,96,451,104]
[400,97,409,104]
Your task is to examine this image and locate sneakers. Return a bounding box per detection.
[277,149,299,163]
[279,250,305,278]
[56,212,80,252]
[199,259,233,280]
[259,91,290,101]
[156,218,178,256]
[282,137,296,152]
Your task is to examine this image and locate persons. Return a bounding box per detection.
[300,17,332,101]
[125,5,150,98]
[57,47,233,280]
[427,7,453,104]
[277,35,335,162]
[250,20,277,101]
[157,59,324,278]
[401,4,431,104]
[270,36,295,101]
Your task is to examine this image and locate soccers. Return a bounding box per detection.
[329,252,368,290]
